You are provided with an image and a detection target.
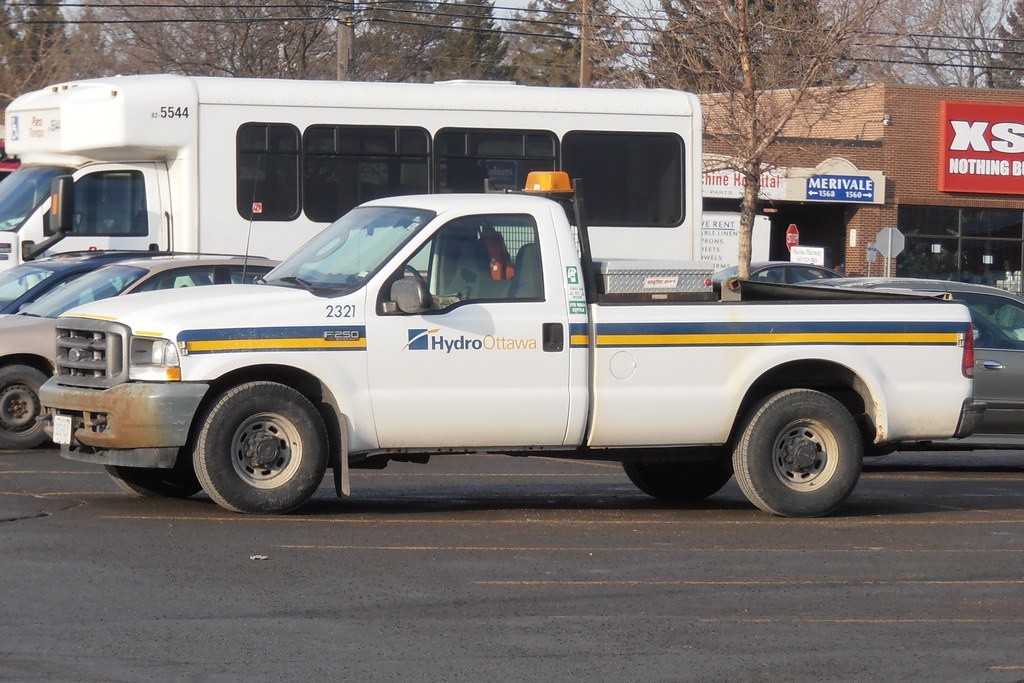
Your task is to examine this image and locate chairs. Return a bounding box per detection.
[446,244,494,302]
[74,201,130,236]
[994,303,1018,342]
[238,176,279,222]
[501,243,544,300]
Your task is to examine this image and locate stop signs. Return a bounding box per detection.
[785,223,799,253]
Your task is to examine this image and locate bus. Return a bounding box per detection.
[0,70,707,293]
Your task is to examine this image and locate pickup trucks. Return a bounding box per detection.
[35,168,990,520]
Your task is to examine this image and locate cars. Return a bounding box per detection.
[0,248,286,453]
[787,275,1024,458]
[709,259,850,299]
